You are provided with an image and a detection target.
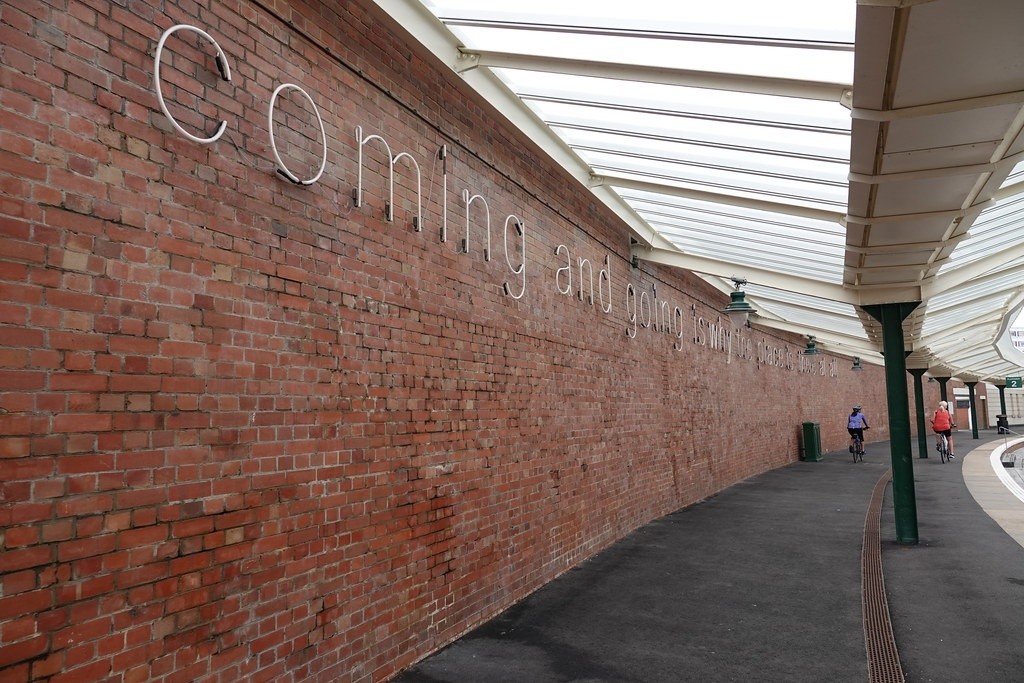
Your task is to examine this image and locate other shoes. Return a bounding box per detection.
[849,445,854,453]
[936,444,941,451]
[949,454,955,458]
[861,450,867,455]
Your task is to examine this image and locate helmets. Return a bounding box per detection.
[852,405,862,410]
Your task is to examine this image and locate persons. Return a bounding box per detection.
[847,405,872,458]
[931,400,956,459]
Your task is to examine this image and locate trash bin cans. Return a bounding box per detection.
[996,415,1009,434]
[801,421,825,463]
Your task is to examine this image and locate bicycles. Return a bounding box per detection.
[847,426,870,463]
[934,423,957,464]
